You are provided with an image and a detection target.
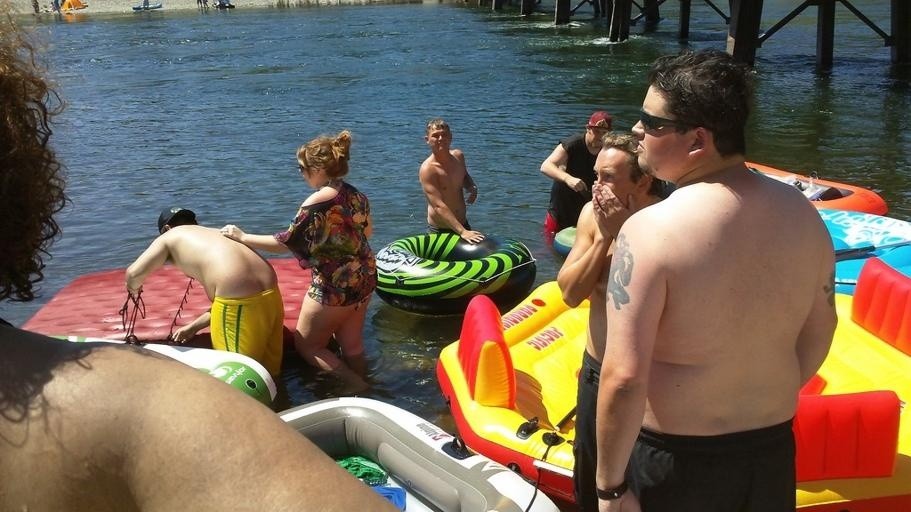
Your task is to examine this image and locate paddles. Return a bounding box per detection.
[833,240,911,260]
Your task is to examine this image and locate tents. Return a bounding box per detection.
[53,0,89,10]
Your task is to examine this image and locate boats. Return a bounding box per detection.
[278,396,563,512]
[212,2,234,8]
[814,208,911,296]
[46,333,278,415]
[743,159,891,219]
[132,2,161,10]
[552,224,578,255]
[436,255,911,511]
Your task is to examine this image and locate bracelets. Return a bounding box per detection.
[596,480,630,499]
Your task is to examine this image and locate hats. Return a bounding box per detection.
[159,205,195,231]
[588,112,613,130]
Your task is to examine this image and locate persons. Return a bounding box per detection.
[419,118,485,245]
[594,43,840,512]
[540,111,614,240]
[125,206,284,377]
[219,129,379,394]
[0,2,399,510]
[559,130,667,510]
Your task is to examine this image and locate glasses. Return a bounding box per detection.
[640,108,688,129]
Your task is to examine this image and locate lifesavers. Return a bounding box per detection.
[553,226,576,256]
